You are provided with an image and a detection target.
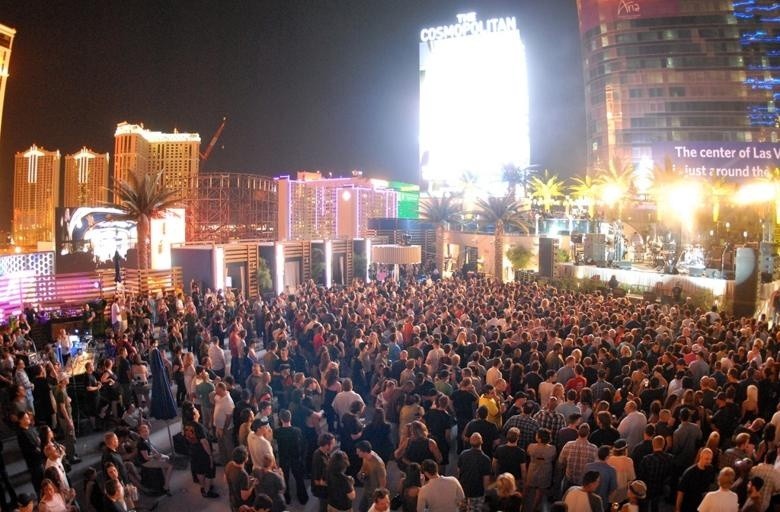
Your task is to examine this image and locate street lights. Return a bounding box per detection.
[731,179,780,280]
[93,281,104,298]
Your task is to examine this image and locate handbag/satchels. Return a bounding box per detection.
[390,494,401,510]
[310,478,327,498]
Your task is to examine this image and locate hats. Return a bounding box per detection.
[251,419,270,431]
[627,479,647,499]
[613,439,627,451]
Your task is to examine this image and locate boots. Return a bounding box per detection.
[201,485,220,498]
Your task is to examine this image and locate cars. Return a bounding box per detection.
[658,239,736,270]
